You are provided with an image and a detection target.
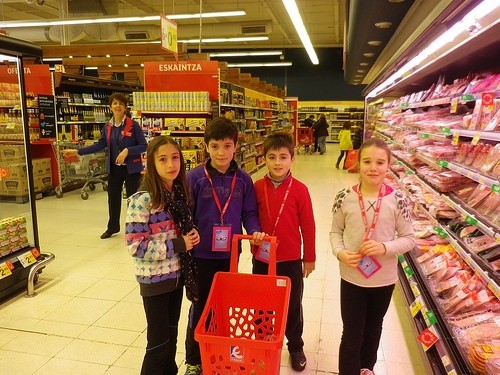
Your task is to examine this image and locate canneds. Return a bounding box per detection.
[133,92,211,112]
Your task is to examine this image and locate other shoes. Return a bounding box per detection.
[183,363,202,375]
[100,228,119,239]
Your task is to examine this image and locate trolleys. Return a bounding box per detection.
[193,233,292,375]
[296,127,317,155]
[48,138,110,200]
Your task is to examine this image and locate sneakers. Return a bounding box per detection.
[288,345,306,372]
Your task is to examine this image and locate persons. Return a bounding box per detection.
[330,137,415,375]
[185,116,268,375]
[352,124,364,149]
[59,93,149,239]
[335,122,353,170]
[250,133,317,372]
[125,136,200,375]
[312,113,329,155]
[303,115,315,151]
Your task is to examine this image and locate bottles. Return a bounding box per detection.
[78,124,102,141]
[0,92,41,141]
[56,106,113,122]
[55,91,110,104]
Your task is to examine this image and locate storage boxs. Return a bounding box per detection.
[0,144,51,197]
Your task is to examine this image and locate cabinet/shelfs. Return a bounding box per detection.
[363,0,500,375]
[218,79,294,175]
[298,110,364,143]
[51,71,144,189]
[130,111,212,175]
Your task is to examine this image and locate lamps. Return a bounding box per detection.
[282,0,319,65]
[0,10,298,67]
